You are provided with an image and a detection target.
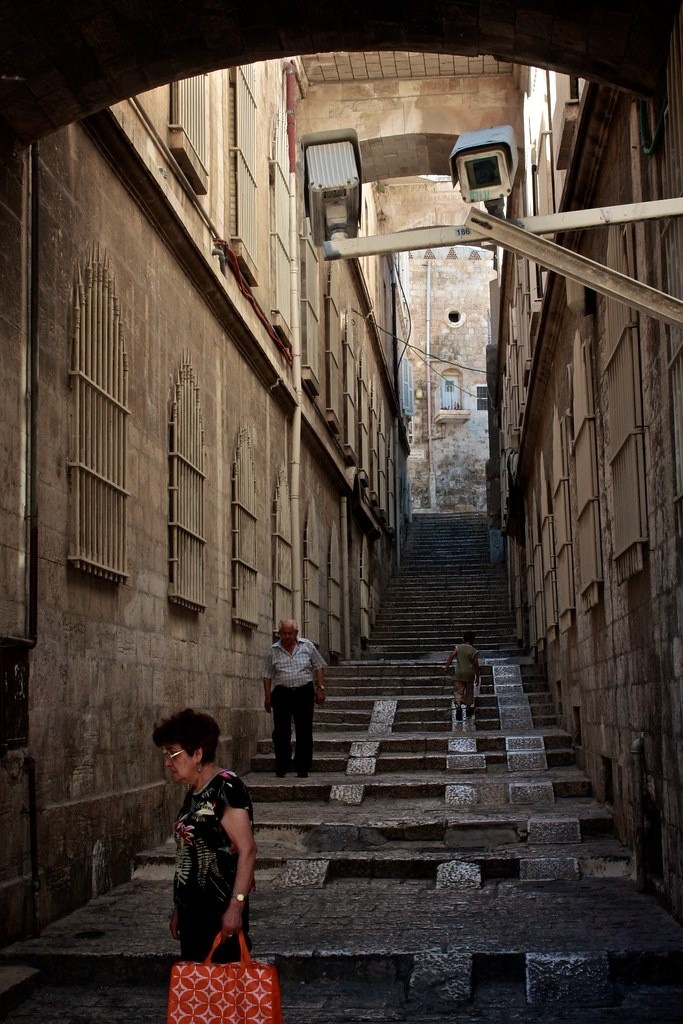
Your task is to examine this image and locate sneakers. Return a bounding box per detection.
[456,707,462,720]
[466,706,474,717]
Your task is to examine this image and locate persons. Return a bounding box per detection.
[444,631,479,721]
[261,620,327,779]
[151,709,258,964]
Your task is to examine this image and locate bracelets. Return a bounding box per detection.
[171,907,177,912]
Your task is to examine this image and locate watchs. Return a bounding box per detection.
[318,685,325,689]
[231,893,247,901]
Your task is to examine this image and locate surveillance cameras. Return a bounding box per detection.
[300,129,363,249]
[447,124,520,203]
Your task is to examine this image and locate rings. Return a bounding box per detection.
[228,935,232,937]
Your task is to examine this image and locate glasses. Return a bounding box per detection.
[162,749,185,761]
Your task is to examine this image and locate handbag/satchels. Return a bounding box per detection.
[166,929,285,1024]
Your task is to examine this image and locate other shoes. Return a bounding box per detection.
[298,769,308,777]
[275,767,287,776]
